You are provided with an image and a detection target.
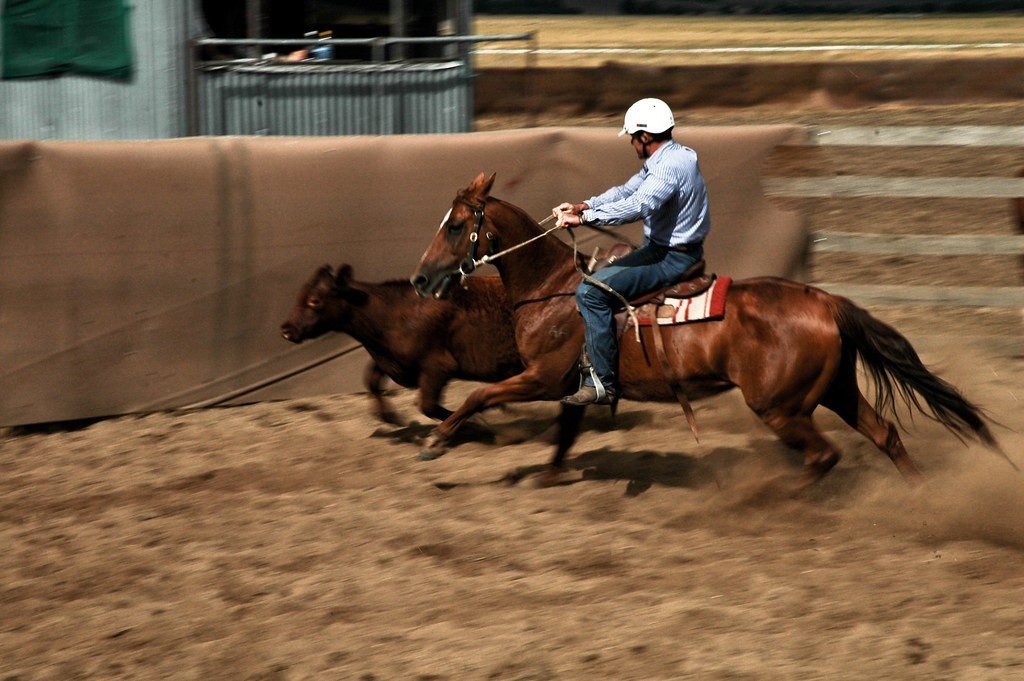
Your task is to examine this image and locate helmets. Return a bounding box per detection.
[618,97,674,137]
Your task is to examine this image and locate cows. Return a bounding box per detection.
[280,263,525,443]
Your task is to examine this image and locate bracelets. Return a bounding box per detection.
[577,211,584,224]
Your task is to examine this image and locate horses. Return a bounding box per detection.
[409,170,1022,489]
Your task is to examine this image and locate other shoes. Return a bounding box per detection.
[561,386,619,407]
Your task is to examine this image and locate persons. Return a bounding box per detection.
[552,97,712,407]
